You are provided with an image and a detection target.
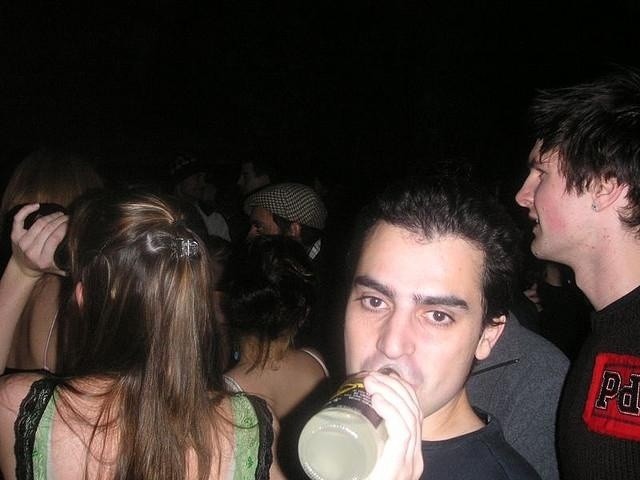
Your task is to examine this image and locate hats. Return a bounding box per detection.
[239,179,331,233]
[164,146,210,194]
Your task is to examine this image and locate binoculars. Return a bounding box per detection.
[9,201,94,272]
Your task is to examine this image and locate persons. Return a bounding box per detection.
[1,74,640,479]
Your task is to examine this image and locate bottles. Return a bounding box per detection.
[297,366,402,480]
[7,202,75,274]
